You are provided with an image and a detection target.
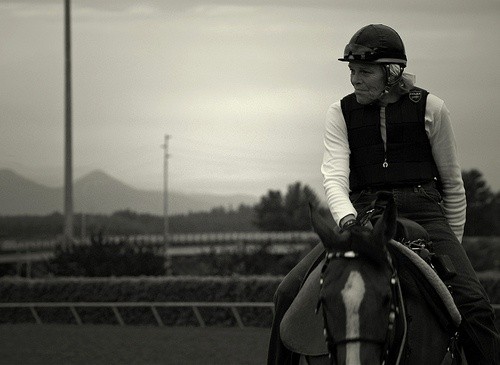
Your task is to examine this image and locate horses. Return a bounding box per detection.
[281,193,462,364]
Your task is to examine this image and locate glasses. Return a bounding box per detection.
[344,42,380,62]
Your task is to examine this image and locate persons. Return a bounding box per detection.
[267,24,500,365]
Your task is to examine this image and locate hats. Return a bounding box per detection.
[337,23,407,104]
